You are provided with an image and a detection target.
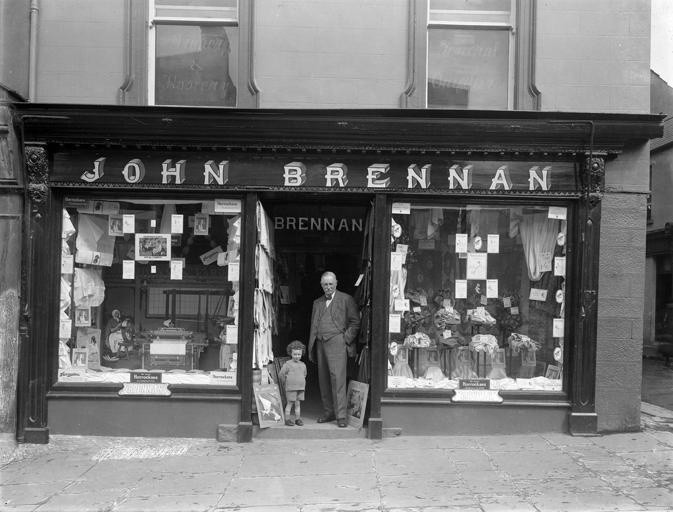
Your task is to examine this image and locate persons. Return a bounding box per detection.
[277,340,308,426]
[105,309,134,358]
[308,271,360,428]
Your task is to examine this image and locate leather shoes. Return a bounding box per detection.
[337,418,348,427]
[318,415,336,423]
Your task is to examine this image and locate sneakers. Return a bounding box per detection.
[295,418,304,426]
[285,419,294,426]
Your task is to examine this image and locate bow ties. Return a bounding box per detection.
[323,296,332,301]
